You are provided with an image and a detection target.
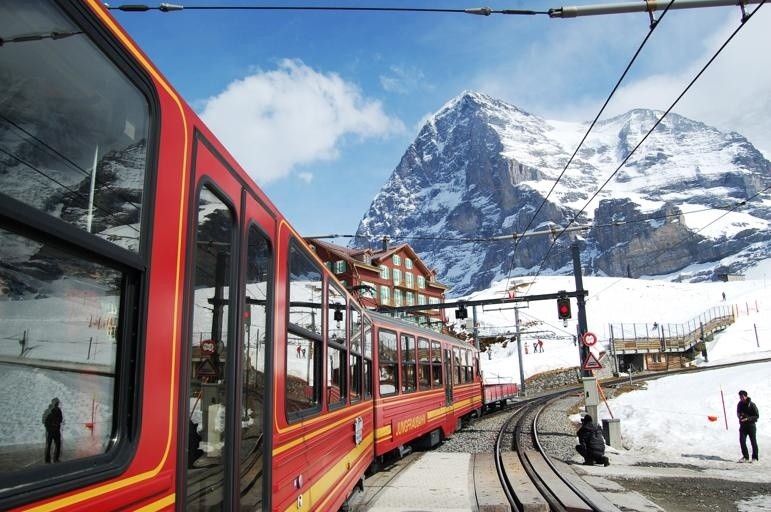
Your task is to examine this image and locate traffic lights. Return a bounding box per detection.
[557,299,571,319]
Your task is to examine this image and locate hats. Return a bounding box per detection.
[581,415,592,422]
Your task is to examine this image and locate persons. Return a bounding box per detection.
[574,335,577,346]
[737,390,761,463]
[575,413,611,467]
[189,418,205,468]
[537,339,545,353]
[487,348,493,360]
[42,397,66,463]
[525,341,530,354]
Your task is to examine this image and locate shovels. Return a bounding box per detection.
[592,369,630,451]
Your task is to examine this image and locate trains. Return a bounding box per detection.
[0,0,520,512]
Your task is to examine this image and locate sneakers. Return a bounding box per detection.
[582,457,610,467]
[738,457,758,464]
